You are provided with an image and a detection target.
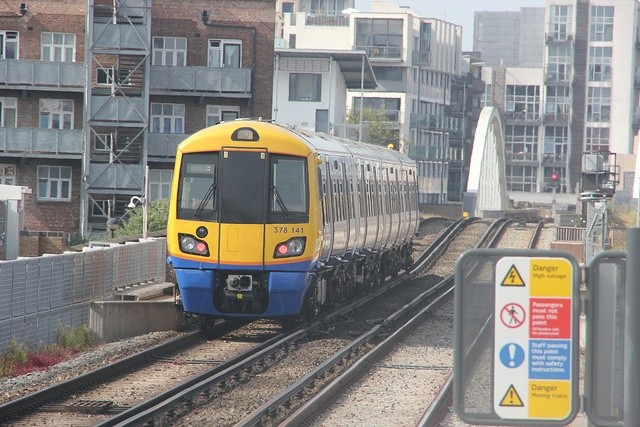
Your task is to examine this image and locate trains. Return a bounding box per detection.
[167,117,420,332]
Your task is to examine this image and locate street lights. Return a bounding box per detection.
[128,196,148,240]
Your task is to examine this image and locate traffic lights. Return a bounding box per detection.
[550,171,559,186]
